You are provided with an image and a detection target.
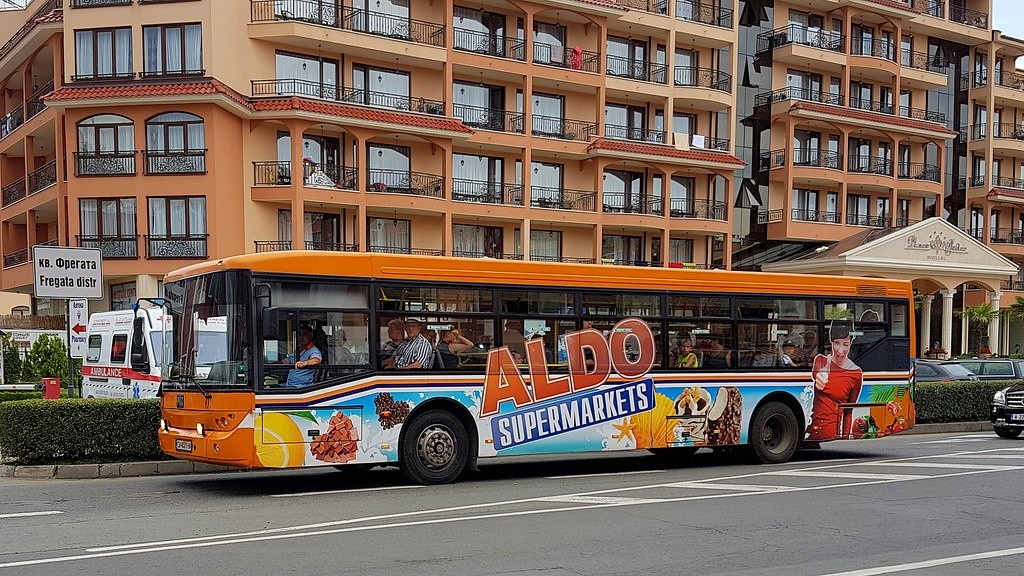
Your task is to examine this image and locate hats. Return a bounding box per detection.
[505,320,520,327]
[782,340,796,347]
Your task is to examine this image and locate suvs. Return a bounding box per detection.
[989,379,1024,437]
[915,358,981,382]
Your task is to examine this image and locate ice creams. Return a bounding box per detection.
[674,386,711,445]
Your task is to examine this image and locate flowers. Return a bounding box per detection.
[980,344,992,354]
[924,347,948,357]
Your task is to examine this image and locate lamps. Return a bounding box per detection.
[376,1,381,7]
[692,38,696,54]
[40,146,45,163]
[556,83,559,100]
[393,210,554,238]
[480,0,485,14]
[304,140,309,149]
[479,145,482,161]
[35,215,40,231]
[460,86,465,95]
[461,156,464,166]
[480,71,484,88]
[302,59,307,70]
[534,96,539,106]
[33,74,38,91]
[459,17,463,23]
[603,174,607,181]
[534,163,539,174]
[807,2,1005,209]
[378,72,382,82]
[556,12,560,29]
[377,148,383,157]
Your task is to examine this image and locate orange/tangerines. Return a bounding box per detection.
[250,411,306,469]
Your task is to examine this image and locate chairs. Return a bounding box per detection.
[740,353,754,366]
[312,327,329,383]
[538,197,553,208]
[751,352,778,366]
[428,349,436,368]
[280,10,295,20]
[435,348,445,368]
[670,209,684,218]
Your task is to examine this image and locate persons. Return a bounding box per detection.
[437,324,473,369]
[502,318,554,365]
[380,318,437,369]
[805,320,862,441]
[653,336,675,370]
[269,325,322,388]
[773,325,818,368]
[671,332,731,370]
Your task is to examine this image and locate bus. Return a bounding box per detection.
[133,249,916,485]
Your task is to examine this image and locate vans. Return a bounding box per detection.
[950,358,1023,380]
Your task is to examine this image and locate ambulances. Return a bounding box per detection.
[82,308,228,399]
